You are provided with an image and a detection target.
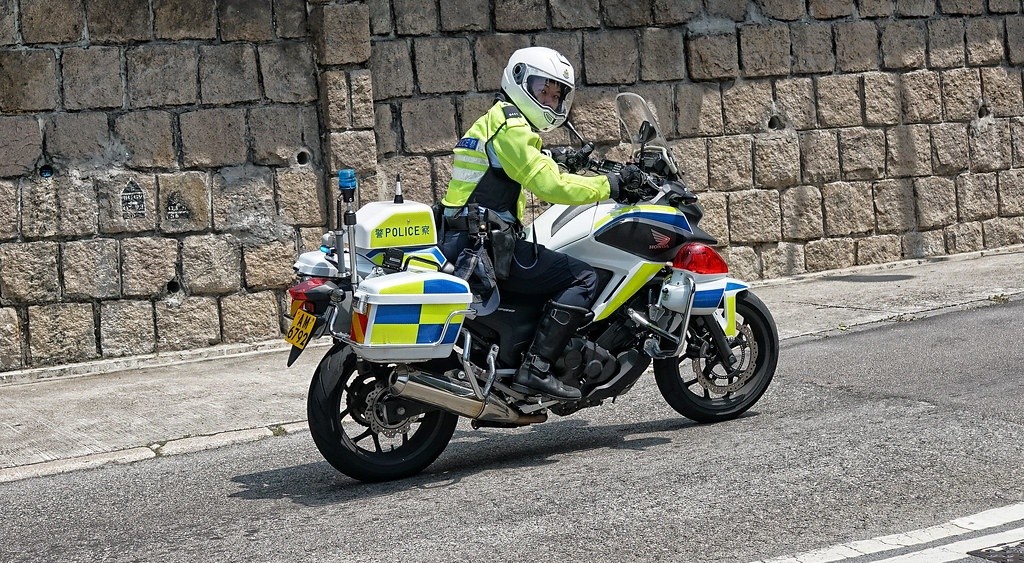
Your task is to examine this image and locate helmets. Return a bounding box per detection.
[501,46,575,133]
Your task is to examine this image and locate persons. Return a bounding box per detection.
[440,46,642,400]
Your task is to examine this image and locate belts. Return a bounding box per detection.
[437,213,493,232]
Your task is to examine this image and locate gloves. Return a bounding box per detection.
[616,164,645,188]
[551,146,574,163]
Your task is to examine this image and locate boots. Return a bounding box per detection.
[510,298,590,400]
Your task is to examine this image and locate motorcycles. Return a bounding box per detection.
[281,91,779,485]
